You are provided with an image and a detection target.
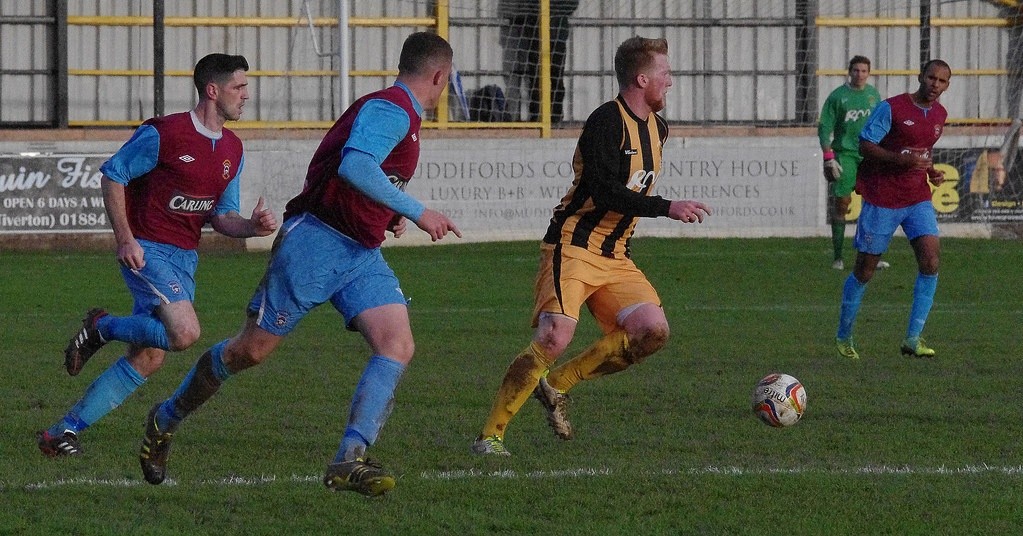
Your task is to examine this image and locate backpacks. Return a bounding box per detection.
[471,85,516,125]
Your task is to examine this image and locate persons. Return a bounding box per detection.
[835,58,952,359]
[816,54,891,273]
[474,34,712,459]
[136,31,464,499]
[35,52,279,460]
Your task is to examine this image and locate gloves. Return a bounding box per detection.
[821,153,843,181]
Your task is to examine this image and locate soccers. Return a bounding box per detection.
[750,374,807,427]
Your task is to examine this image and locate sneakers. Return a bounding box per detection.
[326,451,397,497]
[140,402,174,486]
[898,336,937,361]
[36,424,89,462]
[872,258,889,271]
[833,335,864,366]
[64,305,111,376]
[470,431,515,459]
[832,258,846,272]
[532,370,578,443]
